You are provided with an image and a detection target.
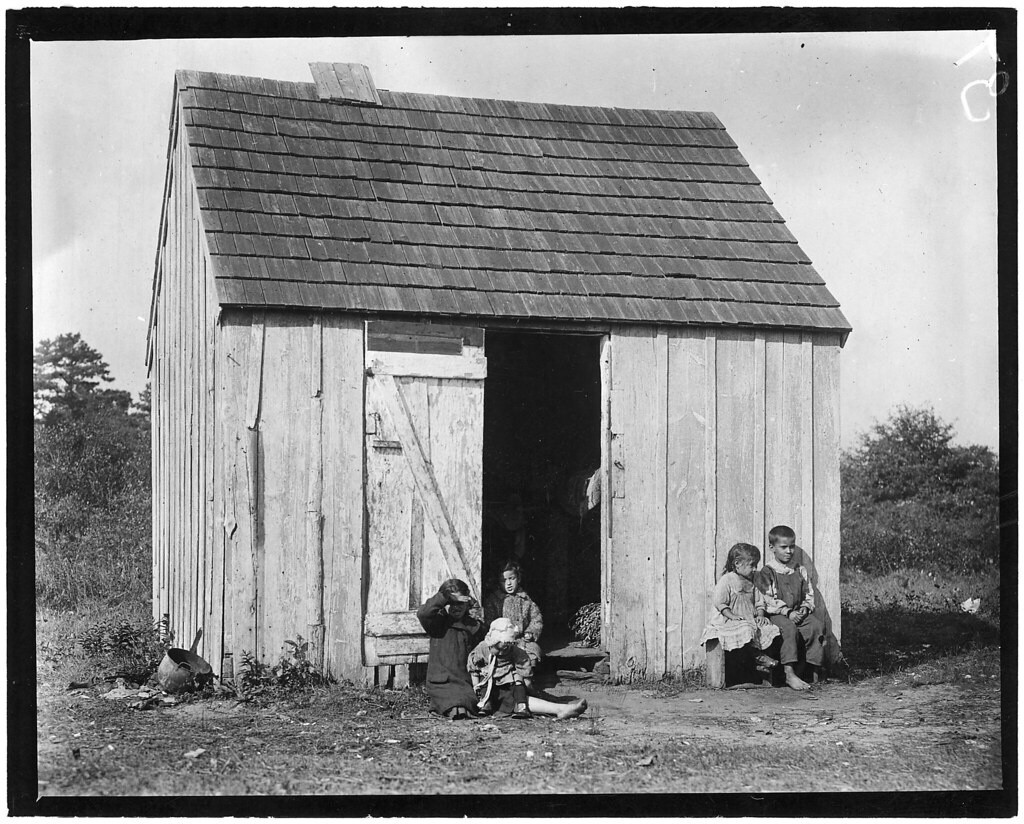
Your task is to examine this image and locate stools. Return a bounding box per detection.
[705,629,811,691]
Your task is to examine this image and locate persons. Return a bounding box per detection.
[464,617,534,718]
[700,542,781,690]
[502,560,544,696]
[416,577,588,719]
[753,525,827,691]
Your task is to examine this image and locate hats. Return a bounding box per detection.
[485,618,519,647]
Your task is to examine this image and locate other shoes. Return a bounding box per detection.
[514,703,529,715]
[755,653,779,673]
[478,701,493,715]
